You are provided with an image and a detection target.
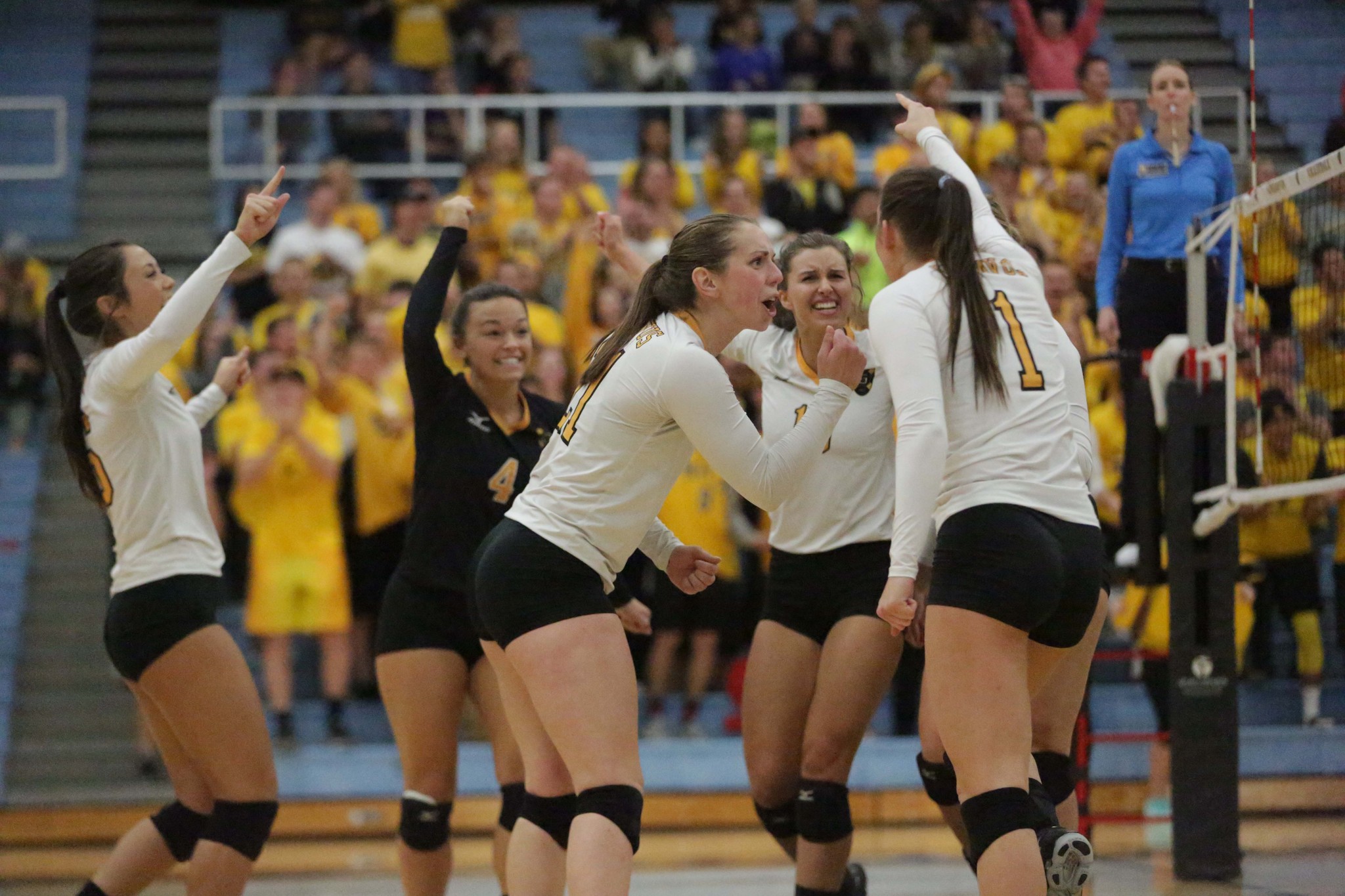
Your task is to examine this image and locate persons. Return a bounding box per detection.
[0,0,1345,896]
[46,164,292,896]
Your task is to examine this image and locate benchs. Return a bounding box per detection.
[0,0,1345,798]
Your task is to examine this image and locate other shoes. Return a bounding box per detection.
[1115,542,1140,564]
[843,862,867,895]
[1038,826,1096,896]
[1301,714,1336,727]
[1143,793,1173,817]
[679,707,704,739]
[643,712,666,740]
